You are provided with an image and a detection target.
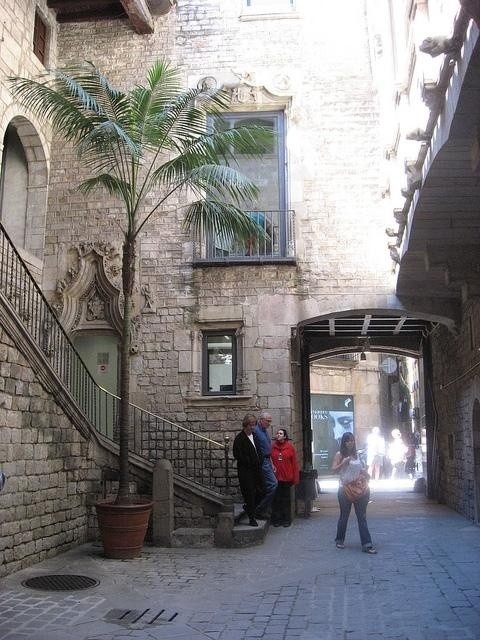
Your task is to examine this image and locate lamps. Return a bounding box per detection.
[360,349,367,361]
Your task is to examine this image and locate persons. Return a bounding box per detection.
[331,432,377,554]
[233,412,299,527]
[247,210,276,255]
[367,427,418,480]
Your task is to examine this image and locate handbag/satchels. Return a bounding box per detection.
[340,473,369,501]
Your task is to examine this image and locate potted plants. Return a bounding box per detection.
[3,53,279,561]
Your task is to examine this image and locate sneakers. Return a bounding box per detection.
[242,503,257,526]
[273,521,291,527]
[335,543,345,549]
[362,546,377,554]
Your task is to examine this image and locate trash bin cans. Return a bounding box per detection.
[297,469,318,519]
